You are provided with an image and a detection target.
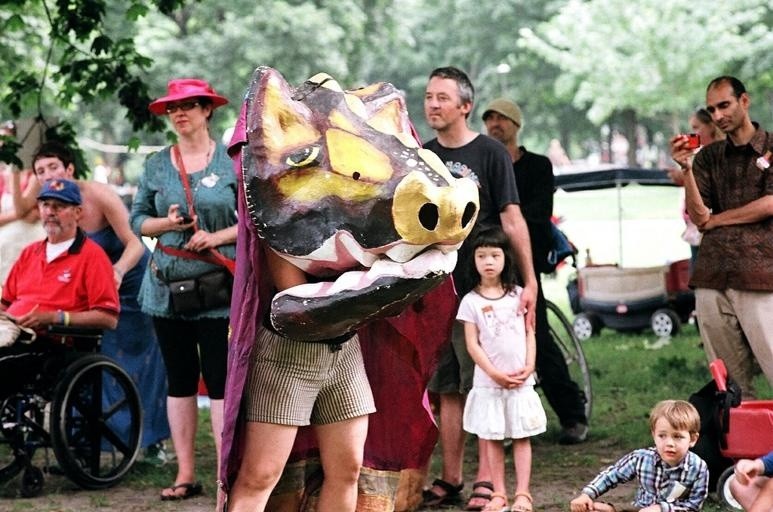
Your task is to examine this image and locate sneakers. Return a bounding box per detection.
[144,440,167,469]
[558,423,588,444]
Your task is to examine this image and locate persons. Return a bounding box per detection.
[131,79,376,512]
[570,400,710,512]
[669,77,773,400]
[418,66,589,511]
[1,142,172,467]
[729,451,773,512]
[669,109,726,348]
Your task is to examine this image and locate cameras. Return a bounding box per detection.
[679,134,700,149]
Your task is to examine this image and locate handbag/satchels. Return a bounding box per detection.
[168,270,233,316]
[542,220,578,275]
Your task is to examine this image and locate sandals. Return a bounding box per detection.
[420,478,532,512]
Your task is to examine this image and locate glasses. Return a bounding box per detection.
[165,98,202,114]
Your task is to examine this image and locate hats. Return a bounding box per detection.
[147,78,227,115]
[481,97,521,128]
[36,179,81,206]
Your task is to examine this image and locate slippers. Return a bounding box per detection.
[160,483,202,501]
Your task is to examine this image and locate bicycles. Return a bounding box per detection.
[530,300,592,448]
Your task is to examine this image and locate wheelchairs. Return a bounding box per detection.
[0,320,144,497]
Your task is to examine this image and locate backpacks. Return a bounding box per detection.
[686,376,741,492]
